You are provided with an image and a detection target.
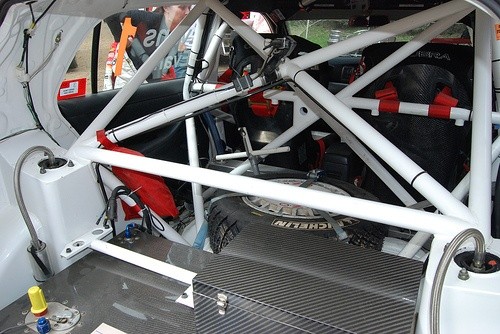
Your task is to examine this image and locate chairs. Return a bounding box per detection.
[226,33,341,171]
[349,39,497,224]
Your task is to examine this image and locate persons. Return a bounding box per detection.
[103,3,191,92]
